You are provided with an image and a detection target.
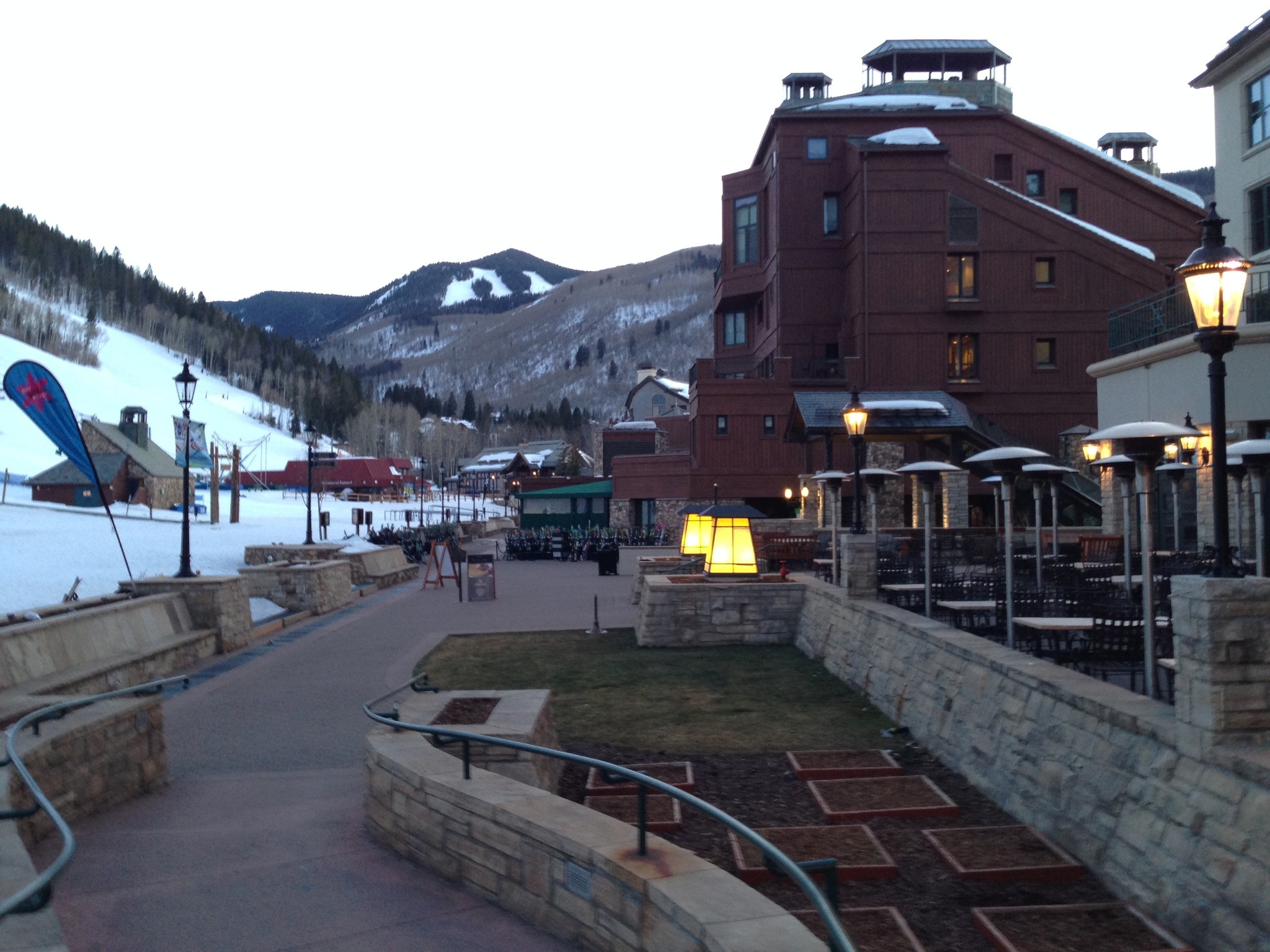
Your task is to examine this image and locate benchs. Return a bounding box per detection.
[762,532,818,574]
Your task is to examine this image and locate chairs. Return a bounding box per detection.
[876,535,1254,702]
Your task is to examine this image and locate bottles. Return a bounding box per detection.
[267,555,272,566]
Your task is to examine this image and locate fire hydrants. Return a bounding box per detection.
[780,561,790,578]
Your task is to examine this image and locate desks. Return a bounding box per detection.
[1053,562,1122,571]
[1011,617,1174,666]
[813,559,839,582]
[935,598,1078,631]
[1085,574,1167,595]
[1003,553,1066,558]
[879,581,994,610]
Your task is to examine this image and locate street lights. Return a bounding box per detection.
[418,456,426,527]
[1173,201,1254,579]
[171,357,200,577]
[456,467,461,523]
[303,420,316,546]
[439,462,446,524]
[471,469,477,520]
[838,390,869,534]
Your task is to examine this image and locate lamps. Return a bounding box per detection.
[801,487,809,507]
[1081,429,1103,476]
[1163,438,1176,464]
[1180,412,1209,469]
[784,488,792,500]
